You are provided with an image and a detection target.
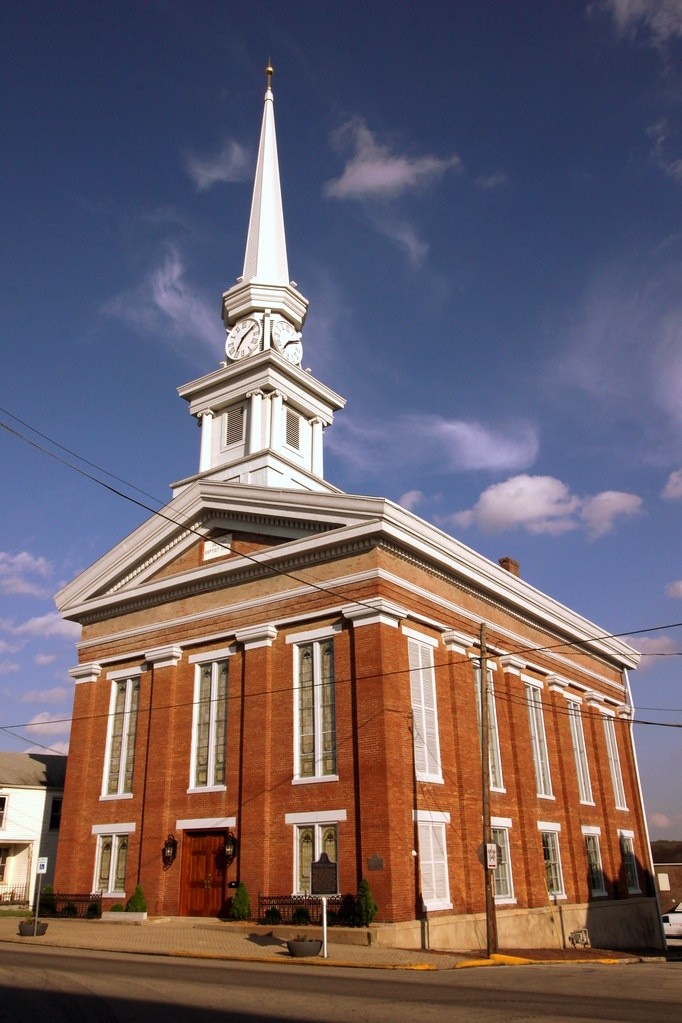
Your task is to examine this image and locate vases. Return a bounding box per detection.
[19,923,48,936]
[286,940,322,957]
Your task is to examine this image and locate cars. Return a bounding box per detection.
[660,901,682,953]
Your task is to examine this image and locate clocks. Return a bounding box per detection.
[226,317,262,360]
[271,321,303,364]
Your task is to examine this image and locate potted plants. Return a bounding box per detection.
[286,935,323,942]
[101,883,147,919]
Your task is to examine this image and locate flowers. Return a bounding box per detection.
[19,918,42,925]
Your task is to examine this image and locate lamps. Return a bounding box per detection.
[163,834,176,860]
[223,832,238,859]
